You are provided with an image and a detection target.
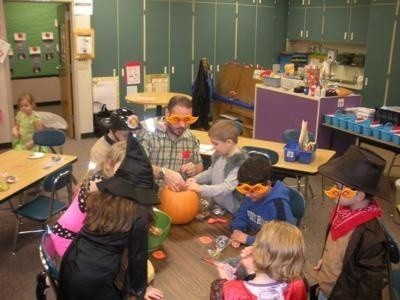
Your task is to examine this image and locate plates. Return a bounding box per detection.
[27,152,44,158]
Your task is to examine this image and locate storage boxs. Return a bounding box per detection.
[359,142,400,179]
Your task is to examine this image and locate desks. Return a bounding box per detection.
[190,129,337,231]
[321,123,400,180]
[322,123,400,149]
[146,217,249,300]
[0,150,77,203]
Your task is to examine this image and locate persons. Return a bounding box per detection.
[210,218,308,300]
[36,141,127,300]
[138,95,204,208]
[313,143,390,300]
[185,119,250,215]
[88,108,142,172]
[11,92,49,195]
[56,131,164,300]
[229,155,297,248]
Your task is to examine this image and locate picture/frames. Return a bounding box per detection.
[73,28,95,60]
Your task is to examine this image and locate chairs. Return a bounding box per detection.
[11,164,72,255]
[244,150,272,168]
[38,246,59,299]
[33,130,66,154]
[282,130,317,200]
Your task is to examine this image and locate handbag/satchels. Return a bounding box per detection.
[93,104,113,136]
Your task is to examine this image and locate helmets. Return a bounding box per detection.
[109,107,143,130]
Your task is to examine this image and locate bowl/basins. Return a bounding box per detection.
[281,79,304,90]
[147,211,171,253]
[262,77,280,86]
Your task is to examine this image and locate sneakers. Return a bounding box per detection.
[36,271,49,299]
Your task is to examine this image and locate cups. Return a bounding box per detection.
[215,234,229,252]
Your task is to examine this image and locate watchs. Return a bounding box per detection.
[160,167,167,180]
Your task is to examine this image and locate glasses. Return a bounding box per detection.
[166,115,199,125]
[323,184,359,200]
[234,184,270,195]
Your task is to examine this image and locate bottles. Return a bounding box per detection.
[357,76,363,88]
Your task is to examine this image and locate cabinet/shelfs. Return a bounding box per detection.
[288,0,400,111]
[91,0,287,120]
[211,99,254,138]
[252,83,363,150]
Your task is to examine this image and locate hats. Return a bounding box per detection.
[96,132,162,205]
[318,144,394,202]
